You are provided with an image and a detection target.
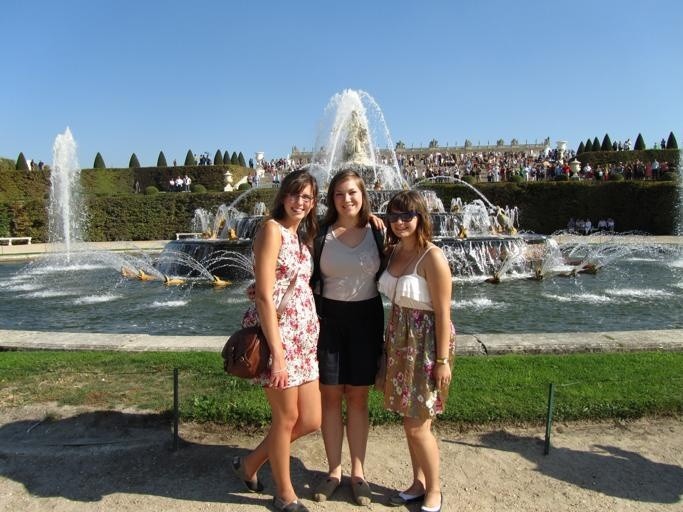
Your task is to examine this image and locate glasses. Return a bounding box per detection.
[388,212,414,222]
[290,194,313,201]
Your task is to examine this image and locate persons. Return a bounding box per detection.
[229,170,385,512]
[368,189,456,512]
[381,150,574,184]
[168,174,191,193]
[247,169,399,505]
[567,216,615,236]
[248,150,328,188]
[583,158,669,181]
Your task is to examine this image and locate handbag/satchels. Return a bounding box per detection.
[222,325,270,378]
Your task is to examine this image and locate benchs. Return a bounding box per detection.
[0,237,32,246]
[568,228,600,234]
[175,232,202,241]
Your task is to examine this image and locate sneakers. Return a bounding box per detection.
[232,456,265,493]
[273,496,309,512]
[390,493,425,506]
[353,481,372,505]
[421,492,443,512]
[314,477,339,502]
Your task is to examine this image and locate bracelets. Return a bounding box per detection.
[436,357,449,365]
